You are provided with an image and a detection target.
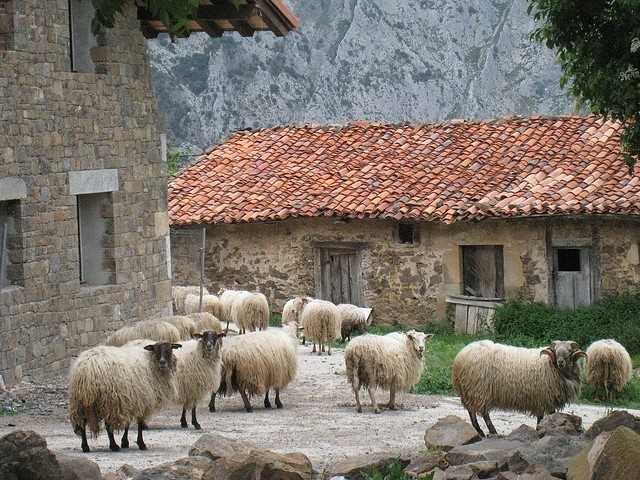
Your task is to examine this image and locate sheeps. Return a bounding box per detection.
[345,329,435,414]
[337,303,368,344]
[584,338,634,403]
[452,339,590,438]
[217,287,270,335]
[121,331,227,430]
[94,320,181,348]
[185,294,221,321]
[209,321,301,412]
[185,312,222,333]
[282,299,306,345]
[292,296,342,357]
[173,285,210,316]
[155,316,196,341]
[67,341,183,453]
